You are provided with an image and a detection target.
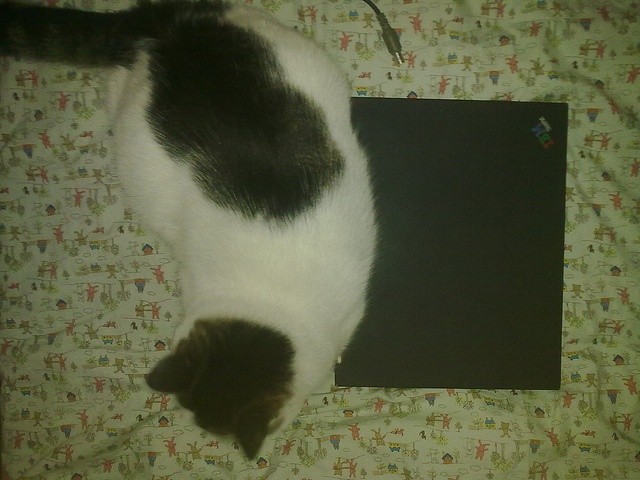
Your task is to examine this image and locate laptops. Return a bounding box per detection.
[333,97,567,390]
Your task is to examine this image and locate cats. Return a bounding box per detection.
[1,0,377,461]
